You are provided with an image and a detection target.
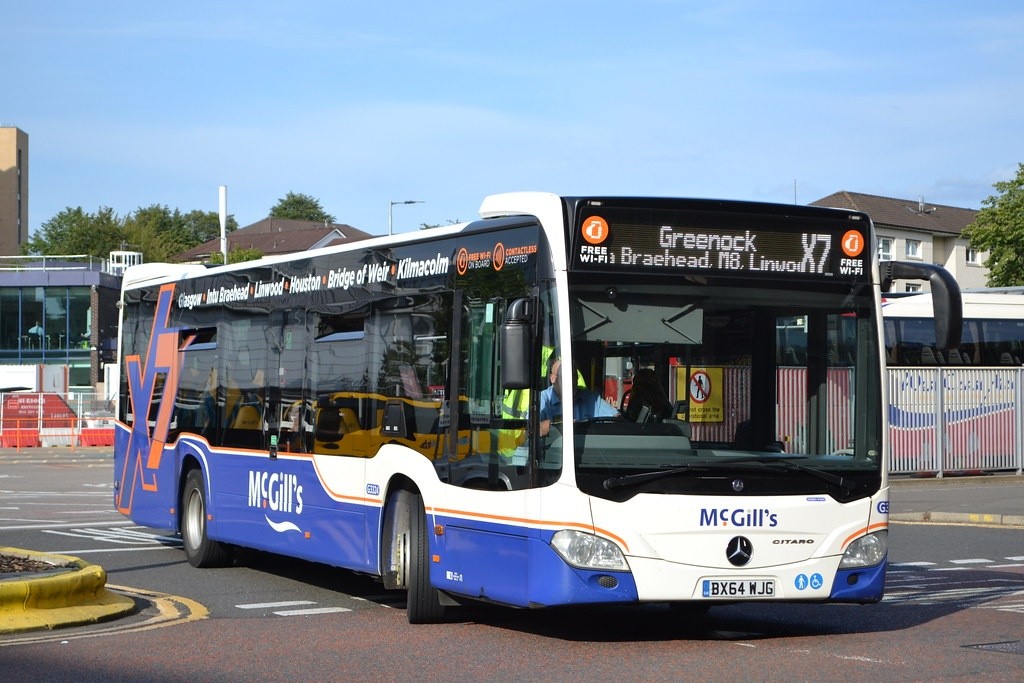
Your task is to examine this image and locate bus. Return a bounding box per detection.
[112,190,963,625]
[621,294,1024,476]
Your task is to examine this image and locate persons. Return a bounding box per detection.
[70,325,92,344]
[27,321,45,349]
[523,348,626,449]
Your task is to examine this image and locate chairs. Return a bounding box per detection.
[786,346,1022,365]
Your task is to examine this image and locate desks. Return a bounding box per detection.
[21,335,51,351]
